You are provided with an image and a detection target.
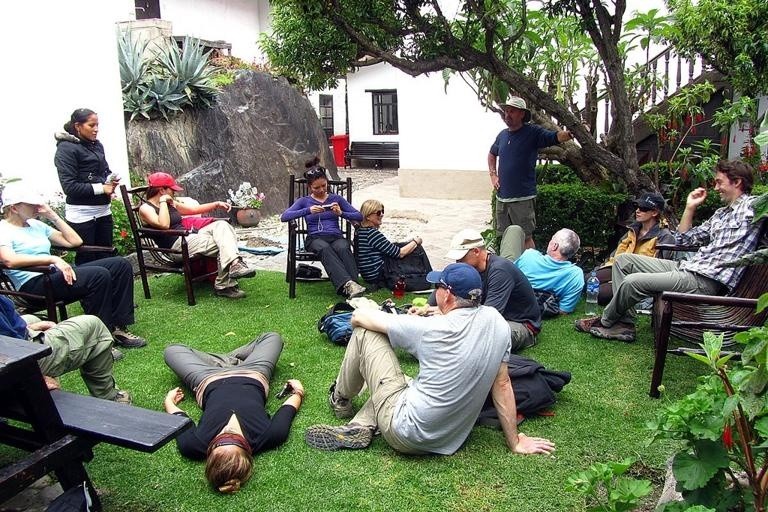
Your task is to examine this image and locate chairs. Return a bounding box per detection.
[0,244,118,324]
[286,175,360,298]
[120,185,232,306]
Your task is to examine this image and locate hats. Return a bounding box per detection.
[426,262,483,300]
[633,192,664,210]
[1,180,43,205]
[498,97,531,122]
[147,172,184,192]
[443,229,486,260]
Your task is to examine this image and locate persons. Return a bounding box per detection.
[354,200,433,289]
[498,224,584,315]
[488,96,590,250]
[581,192,674,306]
[280,164,367,300]
[572,158,768,342]
[303,262,556,457]
[0,180,146,360]
[54,108,139,309]
[426,229,542,353]
[139,172,256,299]
[163,332,305,495]
[0,293,133,406]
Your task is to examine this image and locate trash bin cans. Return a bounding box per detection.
[327,134,351,167]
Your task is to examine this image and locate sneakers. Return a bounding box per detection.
[576,315,601,333]
[304,424,373,450]
[328,380,353,419]
[343,281,366,295]
[228,261,256,279]
[216,287,246,298]
[112,326,146,348]
[590,322,636,343]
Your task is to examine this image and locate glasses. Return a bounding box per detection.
[306,166,322,175]
[633,204,655,212]
[371,209,384,216]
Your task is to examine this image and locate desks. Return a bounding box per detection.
[1,334,102,511]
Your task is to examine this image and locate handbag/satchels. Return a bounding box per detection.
[383,241,432,292]
[295,263,322,278]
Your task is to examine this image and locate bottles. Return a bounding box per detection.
[584,271,599,316]
[392,275,405,299]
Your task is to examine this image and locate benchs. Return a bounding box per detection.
[0,335,193,512]
[344,142,399,170]
[650,220,768,398]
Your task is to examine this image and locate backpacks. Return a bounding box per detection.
[317,303,407,344]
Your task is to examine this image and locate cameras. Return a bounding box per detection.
[322,204,333,211]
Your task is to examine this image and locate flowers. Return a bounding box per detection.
[226,182,266,210]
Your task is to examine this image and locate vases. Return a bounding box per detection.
[237,208,261,228]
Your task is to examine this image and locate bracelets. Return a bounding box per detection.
[568,131,574,140]
[159,201,167,204]
[294,388,305,400]
[412,240,418,245]
